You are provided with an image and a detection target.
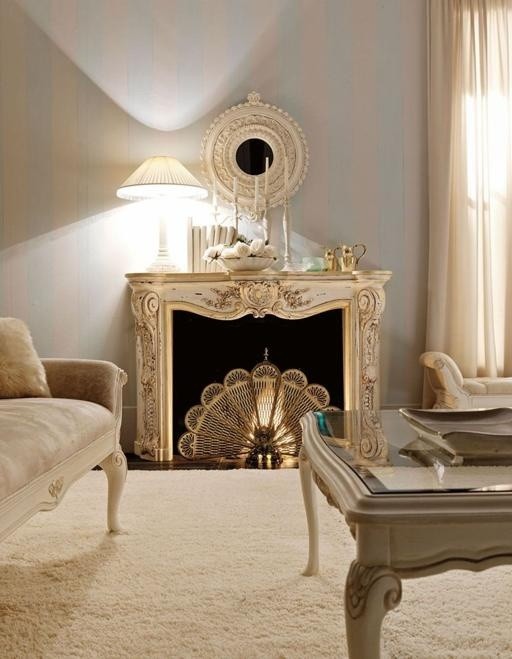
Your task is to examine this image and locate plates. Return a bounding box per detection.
[401,440,512,490]
[395,406,511,462]
[213,257,279,271]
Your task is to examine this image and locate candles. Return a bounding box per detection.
[284,157,289,193]
[233,175,238,201]
[255,176,259,205]
[265,157,269,197]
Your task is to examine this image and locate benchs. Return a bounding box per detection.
[415,348,510,409]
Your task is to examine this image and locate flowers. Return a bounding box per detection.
[201,234,278,264]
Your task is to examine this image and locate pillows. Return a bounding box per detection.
[0,317,49,397]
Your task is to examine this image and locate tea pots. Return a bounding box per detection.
[320,241,366,272]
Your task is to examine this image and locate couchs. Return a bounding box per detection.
[0,316,135,551]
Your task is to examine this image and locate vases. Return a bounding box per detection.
[216,258,273,270]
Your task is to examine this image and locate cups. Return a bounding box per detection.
[301,255,326,272]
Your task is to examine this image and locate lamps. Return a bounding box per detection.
[115,152,207,273]
